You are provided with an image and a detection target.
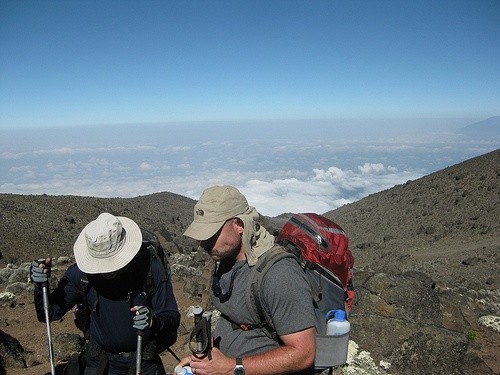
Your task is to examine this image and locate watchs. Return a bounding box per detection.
[233,356,246,375]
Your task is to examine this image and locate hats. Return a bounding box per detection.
[73,212,143,274]
[183,185,276,267]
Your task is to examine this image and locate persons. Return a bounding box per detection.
[30,212,181,375]
[174,185,327,375]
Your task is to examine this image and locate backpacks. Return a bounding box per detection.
[246,212,355,375]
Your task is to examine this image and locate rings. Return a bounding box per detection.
[193,368,196,374]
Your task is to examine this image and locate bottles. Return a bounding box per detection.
[175,365,193,375]
[325,309,350,336]
